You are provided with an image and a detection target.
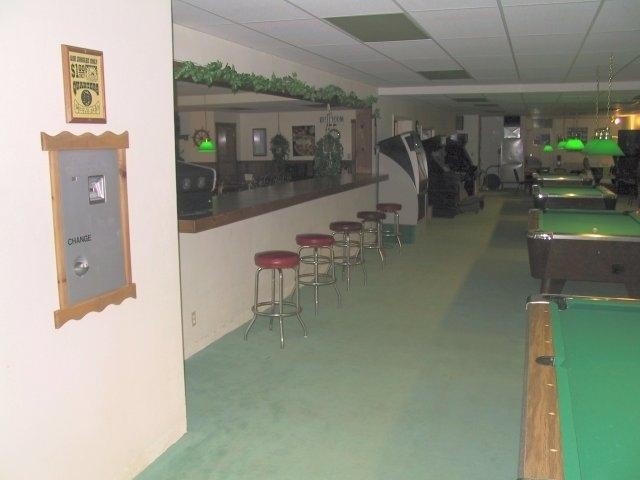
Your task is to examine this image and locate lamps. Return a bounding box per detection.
[197,93,217,152]
[582,51,626,157]
[542,118,553,154]
[564,98,583,151]
[556,114,566,151]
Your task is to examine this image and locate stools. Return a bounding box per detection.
[295,233,341,317]
[376,202,402,249]
[356,211,387,262]
[330,221,369,292]
[244,250,309,349]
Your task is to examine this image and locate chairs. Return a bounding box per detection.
[513,168,530,193]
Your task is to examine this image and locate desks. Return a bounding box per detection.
[530,183,619,210]
[516,289,640,480]
[530,169,593,186]
[523,206,640,298]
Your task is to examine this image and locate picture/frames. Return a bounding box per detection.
[251,127,268,158]
[60,44,106,124]
